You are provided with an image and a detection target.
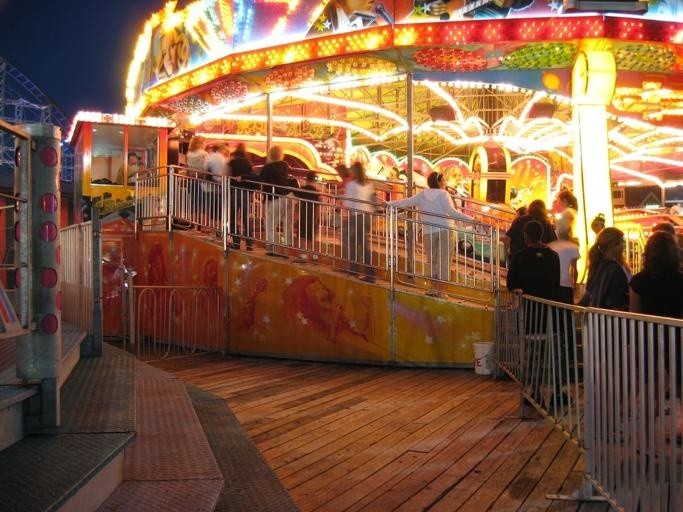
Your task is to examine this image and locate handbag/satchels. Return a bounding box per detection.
[288,178,298,187]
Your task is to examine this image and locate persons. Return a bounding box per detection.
[178,128,322,265]
[338,160,378,284]
[508,191,581,407]
[116,152,152,186]
[576,217,632,443]
[381,171,482,300]
[630,222,682,446]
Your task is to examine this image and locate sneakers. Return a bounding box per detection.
[425,290,448,298]
[550,391,573,406]
[247,244,251,250]
[360,274,376,282]
[523,392,545,408]
[229,241,237,248]
